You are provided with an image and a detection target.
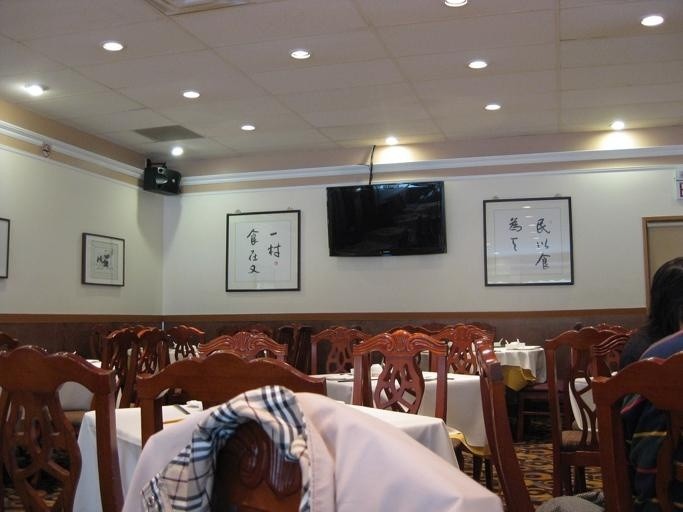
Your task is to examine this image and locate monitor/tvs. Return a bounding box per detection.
[327,181,446,257]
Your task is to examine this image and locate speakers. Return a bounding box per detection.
[145,167,181,195]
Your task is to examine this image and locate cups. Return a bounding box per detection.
[493,342,500,352]
[371,364,382,378]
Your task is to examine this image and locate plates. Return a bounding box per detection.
[316,373,354,380]
[422,370,437,380]
[506,344,540,350]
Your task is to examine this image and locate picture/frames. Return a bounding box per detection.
[81,233,125,287]
[484,196,575,287]
[0,218,10,281]
[226,211,300,292]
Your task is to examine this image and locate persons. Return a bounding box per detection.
[619,254,683,512]
